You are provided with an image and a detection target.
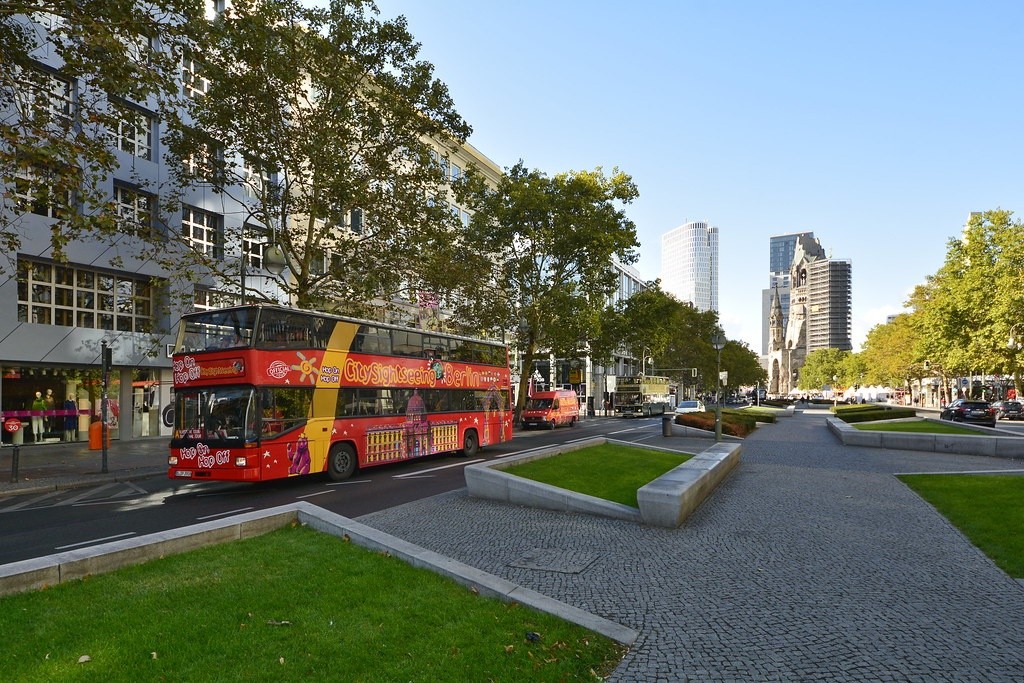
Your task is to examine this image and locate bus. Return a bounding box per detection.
[613,372,671,420]
[165,301,514,484]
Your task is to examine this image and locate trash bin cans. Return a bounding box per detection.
[88,421,110,450]
[662,417,672,436]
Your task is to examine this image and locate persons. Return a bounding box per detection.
[32,389,55,442]
[64,393,77,442]
[217,330,249,348]
[801,396,805,404]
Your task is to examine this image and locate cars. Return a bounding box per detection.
[673,400,707,424]
[991,399,1024,420]
[940,398,996,428]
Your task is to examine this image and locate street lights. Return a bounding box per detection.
[711,333,727,441]
[643,347,654,375]
[240,209,286,306]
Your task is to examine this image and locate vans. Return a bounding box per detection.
[521,388,579,430]
[694,387,770,407]
